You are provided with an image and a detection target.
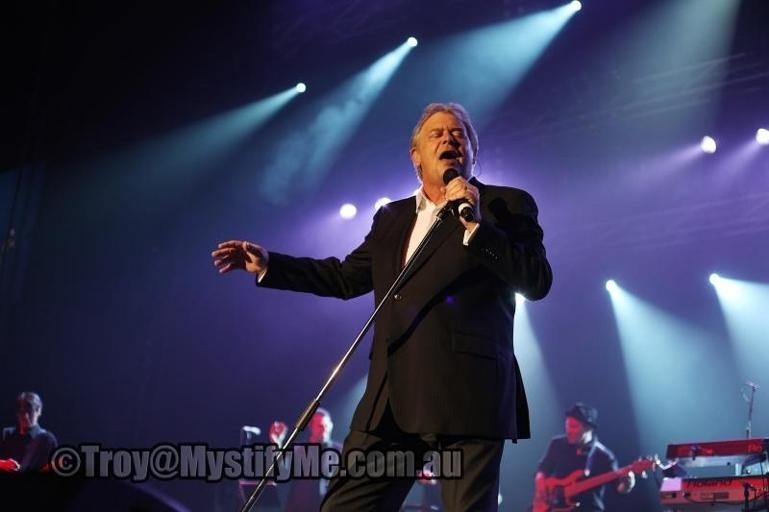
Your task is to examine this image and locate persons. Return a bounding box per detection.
[0,391,64,472]
[209,102,553,511]
[534,401,637,512]
[417,466,503,511]
[288,407,345,511]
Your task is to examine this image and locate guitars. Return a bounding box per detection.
[530,457,655,512]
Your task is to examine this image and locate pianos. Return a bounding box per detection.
[660,438,769,504]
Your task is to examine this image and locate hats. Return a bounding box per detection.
[562,401,600,431]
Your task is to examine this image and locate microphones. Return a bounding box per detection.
[243,425,261,434]
[443,168,474,222]
[747,382,757,390]
